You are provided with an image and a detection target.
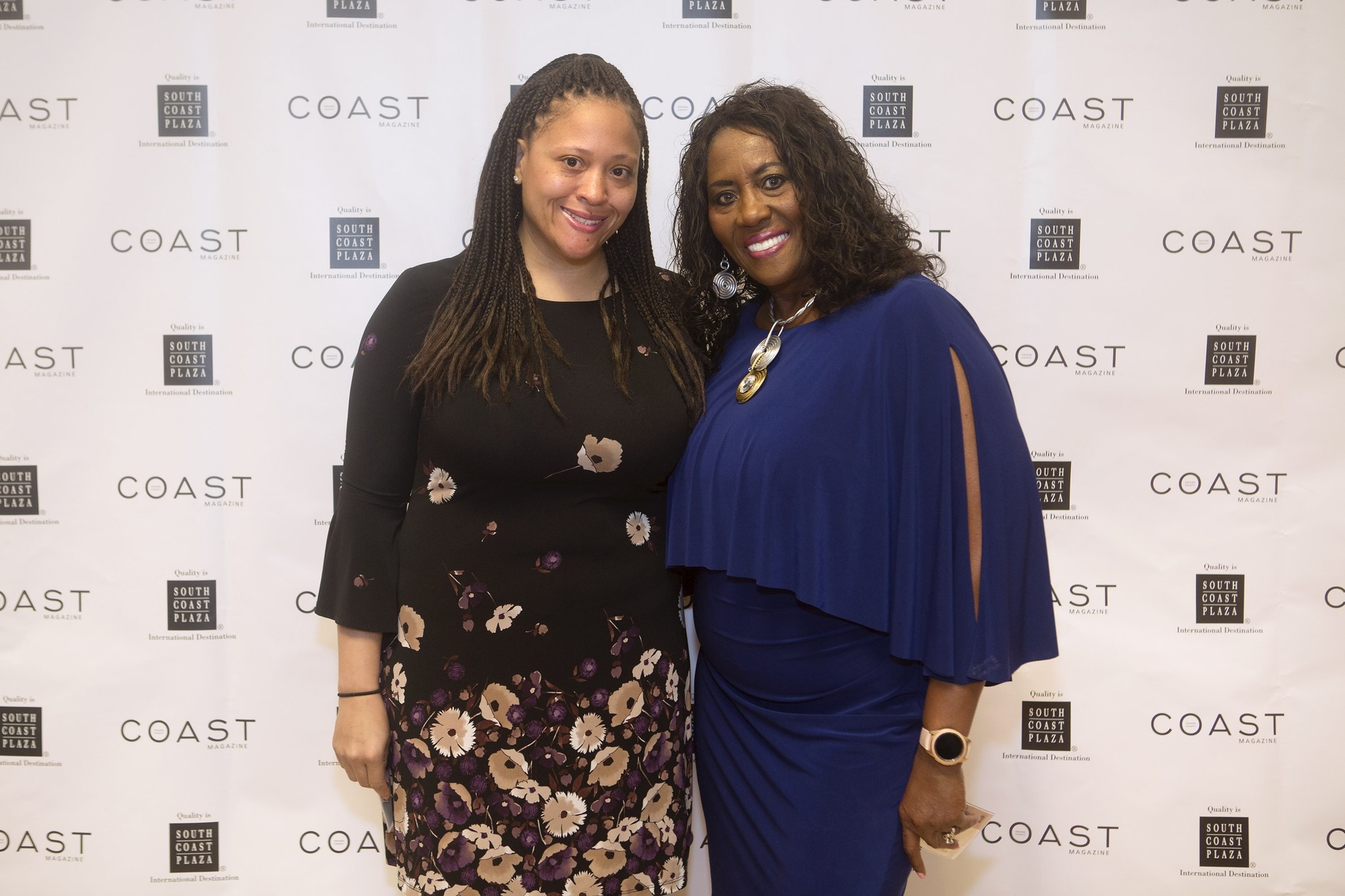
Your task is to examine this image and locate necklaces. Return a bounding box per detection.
[735,289,819,403]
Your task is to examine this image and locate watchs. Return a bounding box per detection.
[919,725,969,768]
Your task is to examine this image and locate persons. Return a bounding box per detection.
[312,54,740,896]
[665,80,1061,896]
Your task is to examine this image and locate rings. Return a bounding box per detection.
[943,827,958,846]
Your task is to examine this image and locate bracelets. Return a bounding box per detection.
[335,689,383,698]
